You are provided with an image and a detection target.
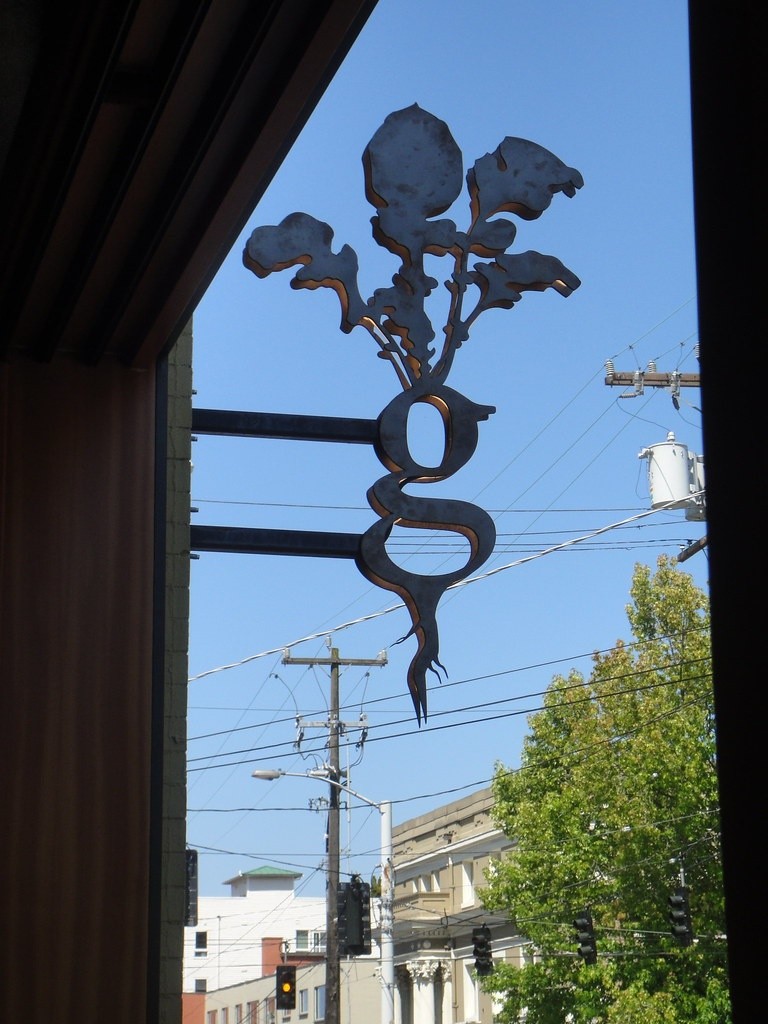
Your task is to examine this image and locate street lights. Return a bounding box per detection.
[253,769,396,1023]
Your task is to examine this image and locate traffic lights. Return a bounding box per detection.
[472,928,493,977]
[574,912,598,965]
[277,967,295,1010]
[668,886,692,946]
